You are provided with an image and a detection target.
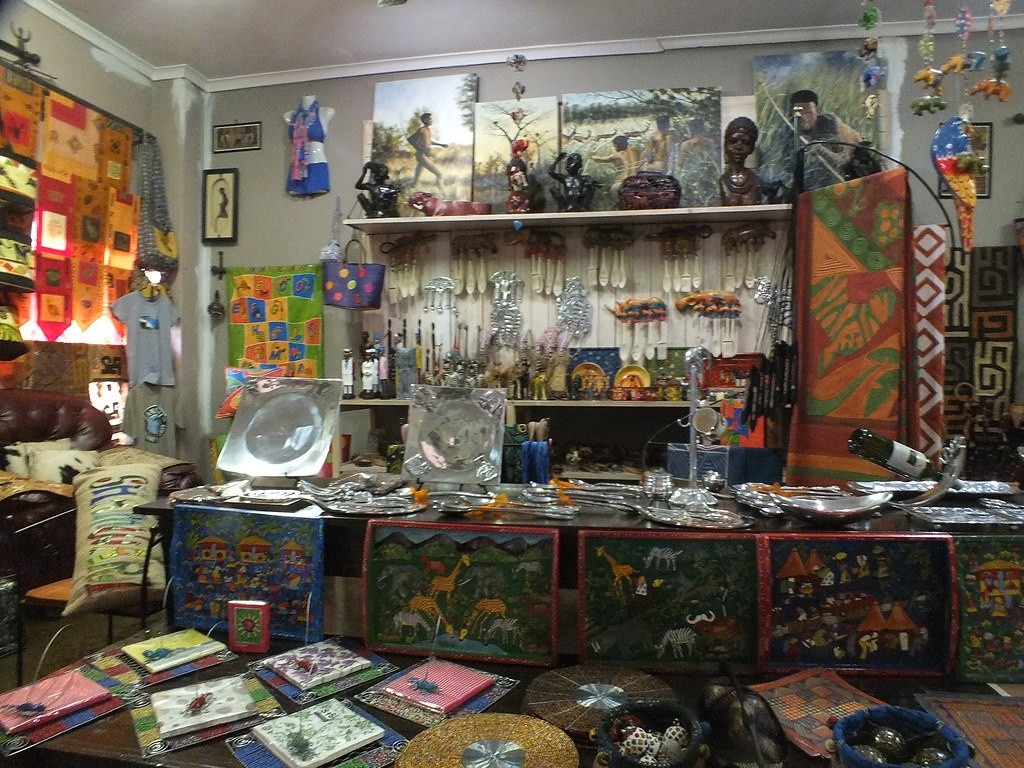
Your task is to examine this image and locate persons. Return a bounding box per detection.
[342,331,389,396]
[283,95,334,196]
[720,117,758,206]
[548,152,596,211]
[507,139,530,214]
[355,164,399,218]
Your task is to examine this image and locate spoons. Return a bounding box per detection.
[432,478,643,520]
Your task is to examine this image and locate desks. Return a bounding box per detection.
[132,477,1024,707]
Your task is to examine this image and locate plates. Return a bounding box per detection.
[769,489,894,527]
[399,383,508,486]
[214,375,344,478]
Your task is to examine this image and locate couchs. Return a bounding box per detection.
[0,388,204,620]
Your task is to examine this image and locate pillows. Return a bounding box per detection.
[61,461,166,619]
[4,436,100,485]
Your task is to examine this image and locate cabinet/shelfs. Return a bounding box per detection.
[340,204,795,408]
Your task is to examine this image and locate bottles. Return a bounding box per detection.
[848,427,965,491]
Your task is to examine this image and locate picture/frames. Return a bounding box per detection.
[212,121,262,153]
[938,122,994,200]
[201,168,238,243]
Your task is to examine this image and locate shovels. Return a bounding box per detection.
[531,253,564,296]
[724,251,755,293]
[618,320,668,361]
[454,257,487,296]
[699,316,735,358]
[388,260,419,304]
[585,247,627,289]
[662,259,702,293]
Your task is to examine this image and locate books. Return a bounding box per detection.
[265,640,370,690]
[254,698,385,768]
[151,676,259,739]
[122,628,227,674]
[381,660,492,714]
[0,671,111,733]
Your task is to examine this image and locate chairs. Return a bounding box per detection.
[12,488,187,687]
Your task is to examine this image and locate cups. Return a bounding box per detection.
[693,406,727,441]
